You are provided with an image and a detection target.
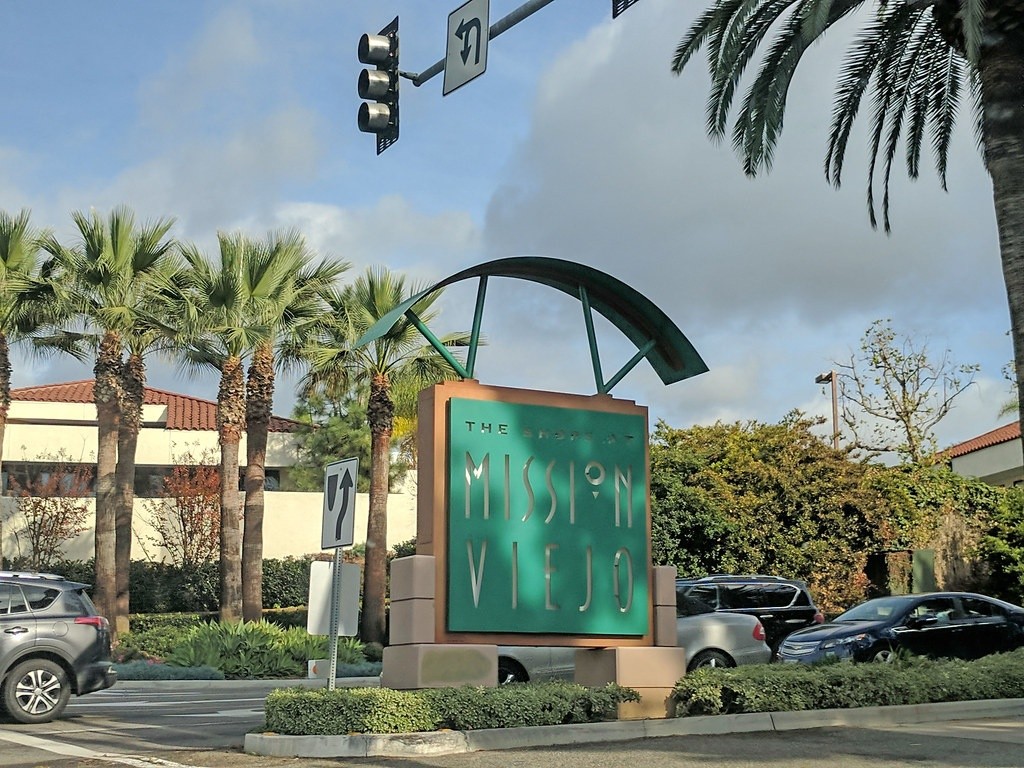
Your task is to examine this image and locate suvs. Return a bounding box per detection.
[675,574,825,665]
[0,571,118,725]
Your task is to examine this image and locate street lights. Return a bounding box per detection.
[815,370,839,451]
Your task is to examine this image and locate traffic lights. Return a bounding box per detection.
[357,15,399,156]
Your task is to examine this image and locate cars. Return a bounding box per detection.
[674,590,771,676]
[777,592,1024,665]
